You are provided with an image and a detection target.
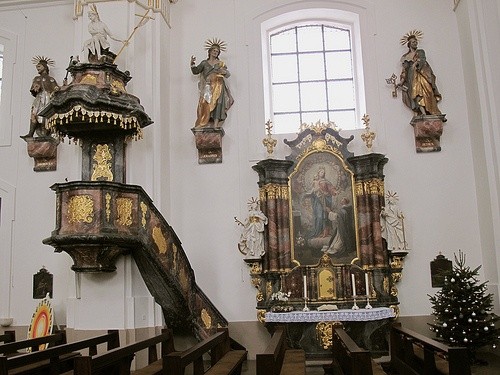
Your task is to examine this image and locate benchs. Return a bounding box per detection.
[0,320,474,375]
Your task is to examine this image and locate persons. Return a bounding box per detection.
[236,197,268,260]
[19,55,62,142]
[379,192,406,250]
[190,38,233,128]
[392,32,448,121]
[82,10,125,60]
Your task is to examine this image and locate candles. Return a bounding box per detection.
[303,275,308,298]
[364,273,369,295]
[351,273,356,295]
